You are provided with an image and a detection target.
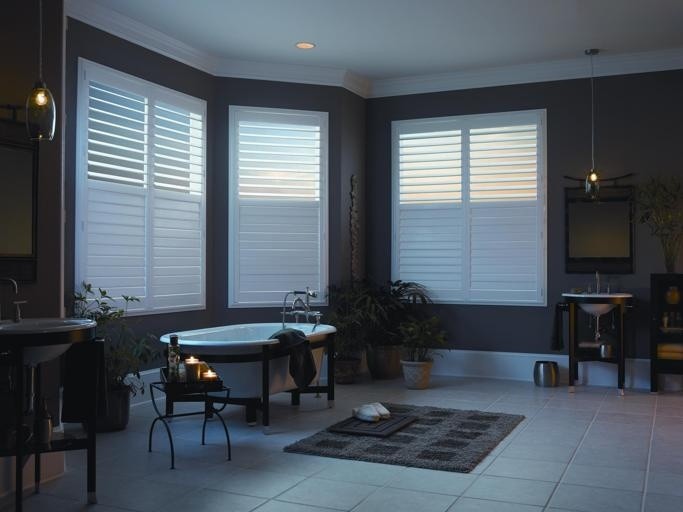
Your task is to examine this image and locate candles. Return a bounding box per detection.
[184,356,219,391]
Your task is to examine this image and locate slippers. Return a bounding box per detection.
[351,404,380,422]
[372,401,392,420]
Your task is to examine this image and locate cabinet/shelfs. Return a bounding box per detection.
[644,267,682,395]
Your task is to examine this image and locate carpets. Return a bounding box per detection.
[281,398,528,475]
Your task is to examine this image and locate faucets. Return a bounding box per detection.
[291,297,305,311]
[0,276,20,295]
[594,270,600,290]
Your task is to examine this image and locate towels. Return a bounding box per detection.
[266,327,319,392]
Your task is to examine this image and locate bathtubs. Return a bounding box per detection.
[160,322,338,402]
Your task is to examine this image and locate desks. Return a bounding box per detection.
[145,366,236,474]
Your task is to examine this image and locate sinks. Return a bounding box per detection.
[0,316,97,365]
[561,292,634,315]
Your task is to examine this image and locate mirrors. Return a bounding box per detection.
[1,116,42,290]
[564,183,638,278]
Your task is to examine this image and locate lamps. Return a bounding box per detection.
[22,0,58,145]
[579,44,605,202]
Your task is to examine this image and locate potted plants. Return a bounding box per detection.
[389,310,451,390]
[323,288,369,385]
[346,266,432,381]
[627,171,683,276]
[67,275,122,382]
[100,291,162,433]
